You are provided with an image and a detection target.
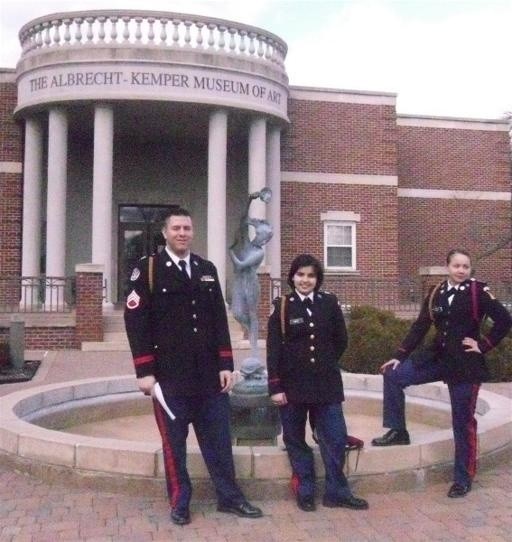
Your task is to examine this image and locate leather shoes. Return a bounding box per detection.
[323,493,369,509]
[448,483,472,498]
[171,510,191,525]
[296,494,315,511]
[372,430,410,446]
[215,501,263,518]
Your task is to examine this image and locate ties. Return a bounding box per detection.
[177,259,191,285]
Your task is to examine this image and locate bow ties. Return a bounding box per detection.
[446,288,457,297]
[302,297,314,312]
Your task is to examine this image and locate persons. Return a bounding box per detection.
[370,249,511,497]
[228,192,274,375]
[123,207,262,523]
[267,254,369,510]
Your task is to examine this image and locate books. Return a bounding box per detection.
[152,381,175,421]
